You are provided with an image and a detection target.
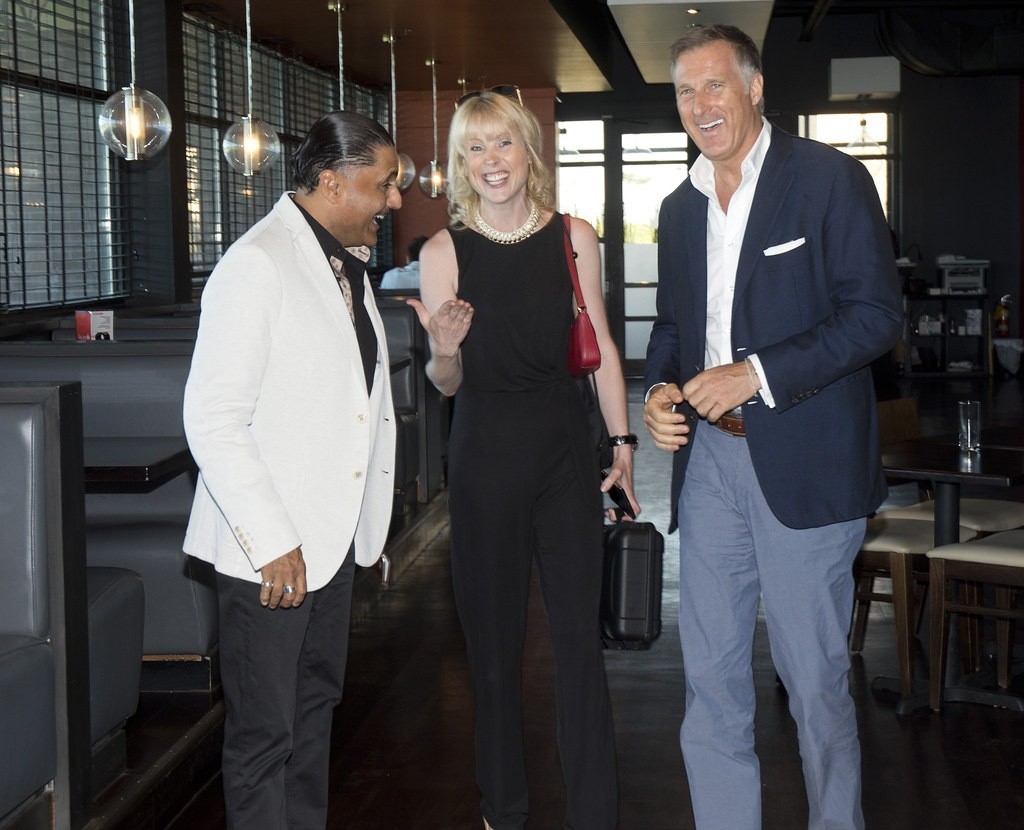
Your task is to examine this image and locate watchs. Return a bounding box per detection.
[611,434,639,451]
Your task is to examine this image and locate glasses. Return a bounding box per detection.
[454,83,524,111]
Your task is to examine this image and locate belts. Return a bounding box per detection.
[713,413,746,436]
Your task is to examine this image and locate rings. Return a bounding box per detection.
[261,581,273,587]
[283,585,296,593]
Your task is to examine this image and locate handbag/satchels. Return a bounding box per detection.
[561,214,601,376]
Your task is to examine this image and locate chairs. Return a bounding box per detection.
[851,397,1024,716]
[0,290,448,830]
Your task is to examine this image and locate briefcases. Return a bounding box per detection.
[599,507,663,651]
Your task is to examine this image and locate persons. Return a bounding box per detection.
[408,94,639,830]
[644,24,903,830]
[381,236,429,289]
[183,112,401,830]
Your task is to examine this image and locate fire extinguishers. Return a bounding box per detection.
[995,294,1013,338]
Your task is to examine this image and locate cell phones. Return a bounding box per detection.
[601,470,636,520]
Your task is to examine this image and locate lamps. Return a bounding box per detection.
[222,0,281,176]
[97,0,171,162]
[382,33,446,198]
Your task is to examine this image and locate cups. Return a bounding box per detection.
[965,308,983,336]
[959,401,982,451]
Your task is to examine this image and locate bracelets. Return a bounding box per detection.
[744,358,756,394]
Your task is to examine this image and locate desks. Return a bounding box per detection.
[885,432,1024,714]
[83,435,188,498]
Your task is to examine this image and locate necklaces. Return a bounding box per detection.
[473,195,539,244]
